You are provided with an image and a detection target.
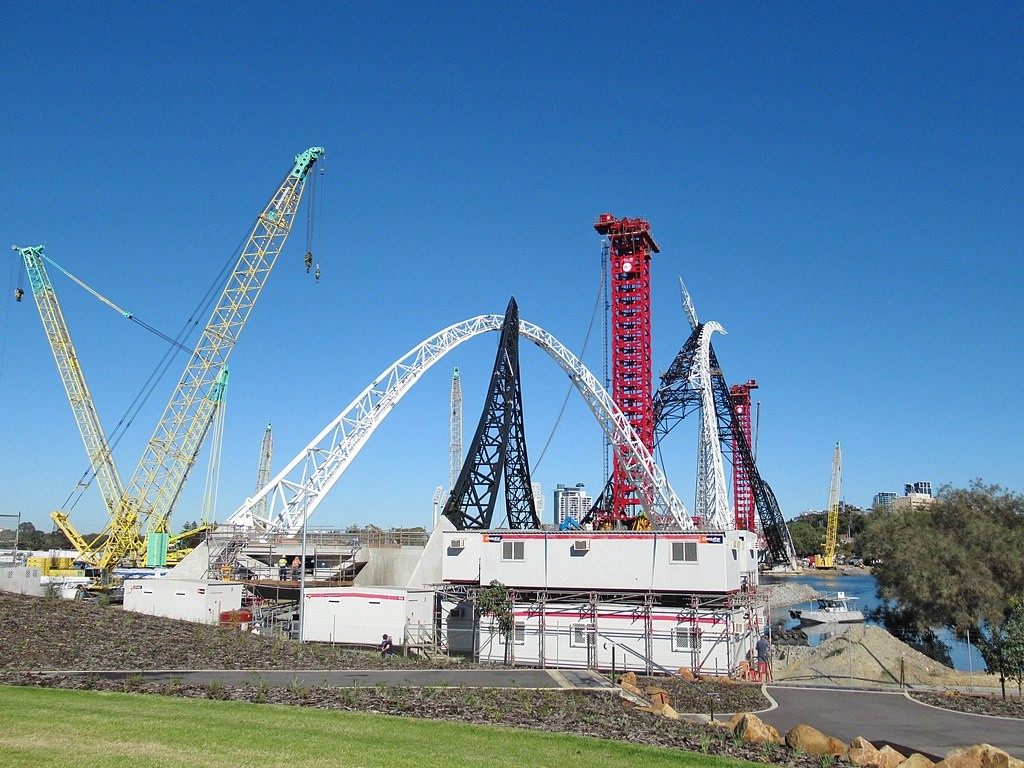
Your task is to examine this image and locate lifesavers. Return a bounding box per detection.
[782,632,790,640]
[800,632,808,639]
[792,631,801,639]
[772,632,781,640]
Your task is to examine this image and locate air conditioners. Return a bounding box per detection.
[731,539,744,549]
[450,539,465,549]
[573,540,591,550]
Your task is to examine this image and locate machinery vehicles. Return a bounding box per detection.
[813,440,843,566]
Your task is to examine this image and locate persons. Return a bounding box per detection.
[380,634,392,657]
[292,557,301,580]
[279,555,288,581]
[755,635,769,672]
[251,624,260,634]
[774,626,782,652]
[350,537,359,553]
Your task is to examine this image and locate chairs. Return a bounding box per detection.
[738,661,756,681]
[755,661,771,683]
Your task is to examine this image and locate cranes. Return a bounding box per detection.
[11,146,328,607]
[433,366,464,530]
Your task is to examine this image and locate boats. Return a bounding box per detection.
[787,591,866,624]
